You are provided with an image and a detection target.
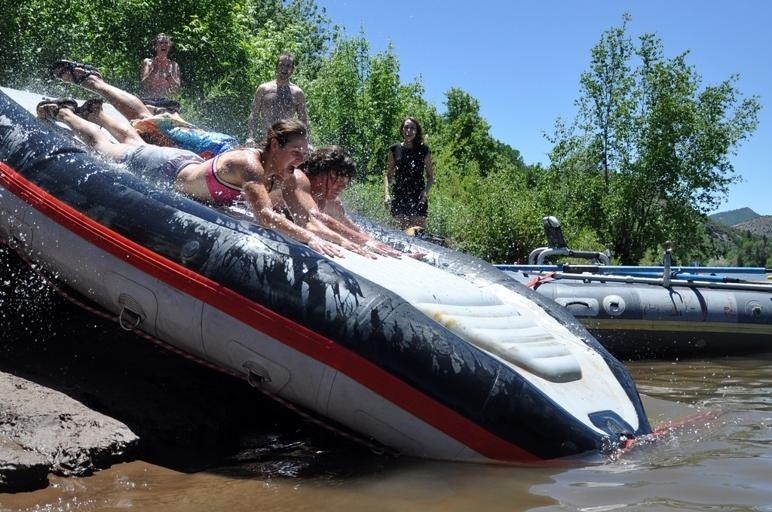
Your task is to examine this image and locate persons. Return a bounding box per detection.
[242,52,315,152]
[34,94,381,264]
[46,56,430,260]
[140,30,182,100]
[380,116,436,230]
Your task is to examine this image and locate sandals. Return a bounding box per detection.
[50,60,102,87]
[36,97,77,120]
[142,97,180,114]
[77,98,104,119]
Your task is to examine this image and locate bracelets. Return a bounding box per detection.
[163,73,172,80]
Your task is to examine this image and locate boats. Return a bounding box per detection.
[1,83,655,471]
[491,215,772,352]
